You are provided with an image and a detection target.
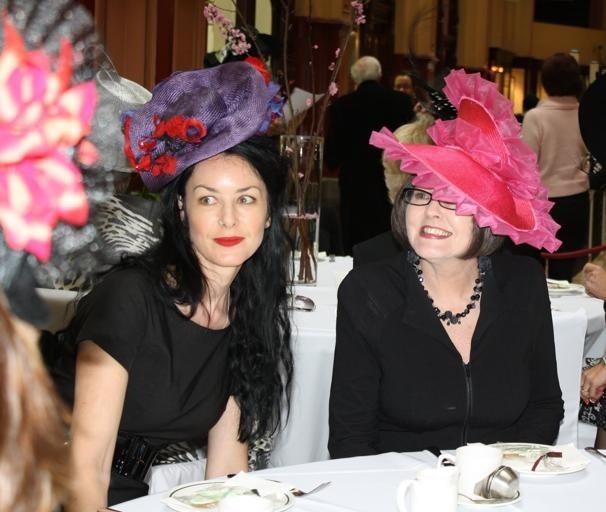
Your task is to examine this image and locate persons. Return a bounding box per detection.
[67,137,292,512]
[326,54,591,282]
[329,175,564,459]
[580,262,606,407]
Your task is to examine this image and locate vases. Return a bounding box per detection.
[278,134,324,287]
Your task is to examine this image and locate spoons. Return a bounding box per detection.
[586,447,606,459]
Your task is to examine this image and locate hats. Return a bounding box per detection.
[128,60,268,192]
[399,98,535,232]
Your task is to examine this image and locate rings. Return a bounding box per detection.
[581,386,589,390]
[589,398,596,404]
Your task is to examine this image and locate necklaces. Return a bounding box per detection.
[414,259,486,325]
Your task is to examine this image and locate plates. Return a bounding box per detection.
[488,443,591,477]
[544,283,586,299]
[164,479,294,512]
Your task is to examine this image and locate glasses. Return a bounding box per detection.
[400,188,458,211]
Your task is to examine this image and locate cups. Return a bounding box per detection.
[437,445,503,493]
[397,470,458,512]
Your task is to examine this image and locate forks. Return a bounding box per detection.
[459,493,506,505]
[228,473,331,498]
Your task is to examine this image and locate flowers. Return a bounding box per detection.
[204,0,367,281]
